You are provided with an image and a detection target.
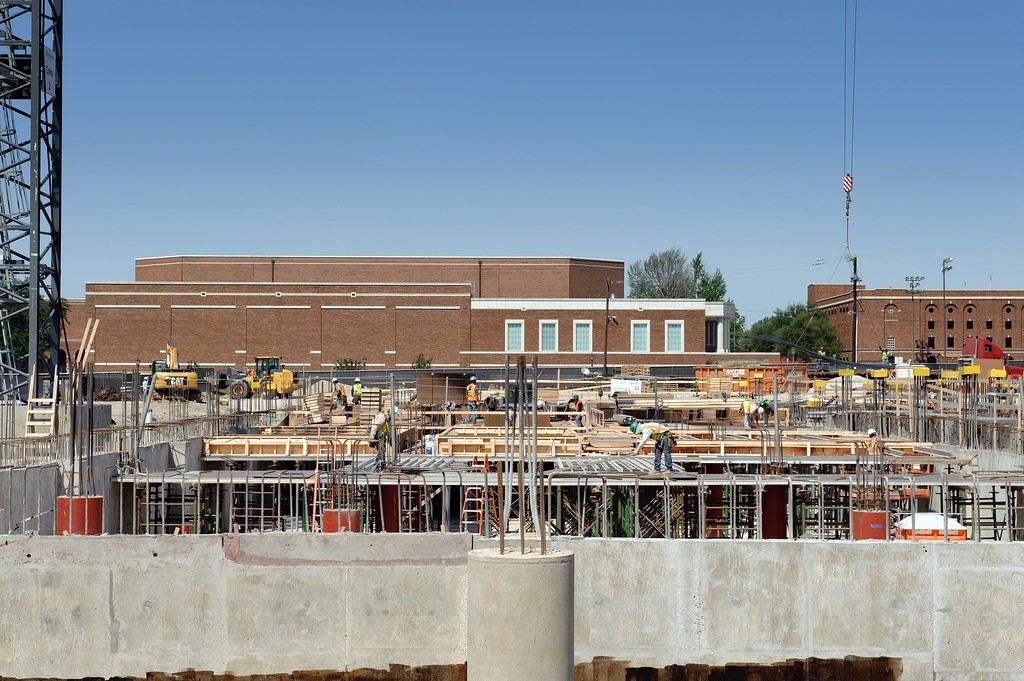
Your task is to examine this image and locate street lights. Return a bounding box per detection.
[791,349,796,363]
[905,276,925,363]
[848,311,858,363]
[941,258,954,363]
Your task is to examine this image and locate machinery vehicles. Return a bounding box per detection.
[204,356,302,400]
[149,341,202,402]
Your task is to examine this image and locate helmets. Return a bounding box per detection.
[355,378,361,382]
[332,378,337,383]
[630,422,638,434]
[574,394,578,399]
[750,404,757,413]
[390,406,398,415]
[470,376,476,381]
[758,407,764,414]
[867,428,876,436]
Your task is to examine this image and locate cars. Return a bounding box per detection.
[120,382,133,393]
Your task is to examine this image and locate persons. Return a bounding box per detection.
[508,406,514,427]
[740,398,774,429]
[351,378,363,405]
[369,405,399,473]
[630,421,674,476]
[332,378,348,420]
[867,429,887,474]
[566,394,584,427]
[882,348,891,366]
[465,376,480,424]
[819,347,826,356]
